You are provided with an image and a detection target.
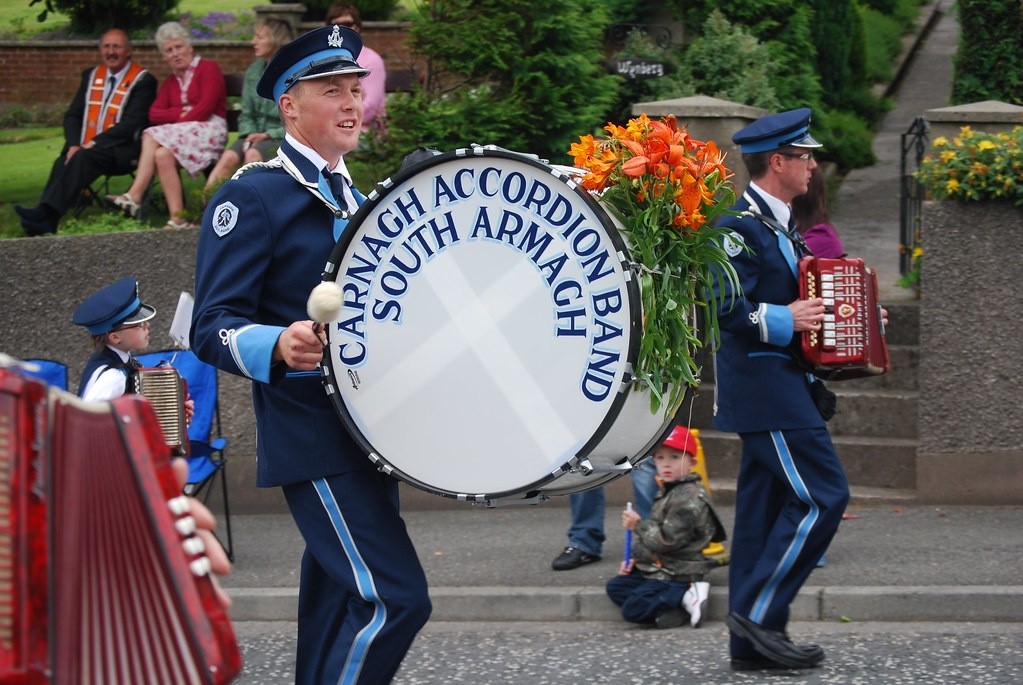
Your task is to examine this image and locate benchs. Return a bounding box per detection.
[135,67,422,214]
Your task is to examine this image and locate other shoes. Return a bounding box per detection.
[14,205,58,236]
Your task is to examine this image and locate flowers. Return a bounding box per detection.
[566,112,757,422]
[912,123,1023,213]
[891,215,924,289]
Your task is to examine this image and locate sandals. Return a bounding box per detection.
[104,191,140,218]
[163,220,189,229]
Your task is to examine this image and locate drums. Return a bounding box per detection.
[310,143,694,508]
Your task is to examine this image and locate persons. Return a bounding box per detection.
[551,457,662,572]
[203,12,294,204]
[711,109,888,669]
[324,2,388,153]
[71,278,195,431]
[605,426,713,628]
[0,354,233,615]
[106,21,226,234]
[15,29,159,238]
[187,25,432,685]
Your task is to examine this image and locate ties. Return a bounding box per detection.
[321,167,348,210]
[101,78,115,116]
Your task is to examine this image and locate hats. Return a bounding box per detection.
[256,23,371,104]
[72,279,157,335]
[732,108,823,154]
[663,424,698,457]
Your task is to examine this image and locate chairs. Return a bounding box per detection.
[8,358,68,391]
[131,348,236,565]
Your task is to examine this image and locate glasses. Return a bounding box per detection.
[109,322,144,333]
[332,21,355,28]
[768,150,814,165]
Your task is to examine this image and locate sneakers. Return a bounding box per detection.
[551,547,598,570]
[682,582,710,628]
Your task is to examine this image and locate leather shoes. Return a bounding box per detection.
[726,611,825,668]
